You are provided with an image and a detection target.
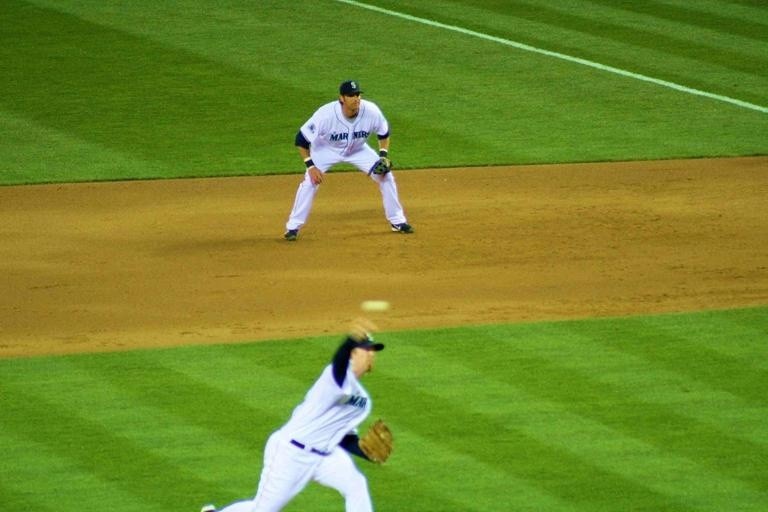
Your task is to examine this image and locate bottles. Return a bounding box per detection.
[339,80,364,95]
[356,333,385,351]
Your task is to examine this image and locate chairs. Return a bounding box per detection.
[284,230,298,242]
[392,222,415,234]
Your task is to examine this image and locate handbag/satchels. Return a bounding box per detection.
[305,158,315,169]
[378,148,388,158]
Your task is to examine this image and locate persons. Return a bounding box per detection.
[200,316,395,512]
[281,81,413,242]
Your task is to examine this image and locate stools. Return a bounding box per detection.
[290,439,331,457]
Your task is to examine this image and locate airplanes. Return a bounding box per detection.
[367,157,392,176]
[358,419,392,463]
